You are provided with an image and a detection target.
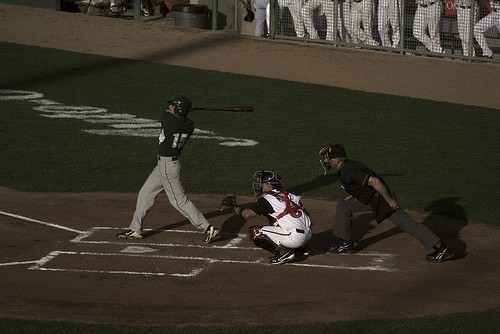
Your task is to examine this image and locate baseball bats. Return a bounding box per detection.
[195,106,253,112]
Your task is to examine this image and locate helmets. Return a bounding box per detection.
[167,94,193,116]
[244,8,255,23]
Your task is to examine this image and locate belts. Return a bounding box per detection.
[296,228,306,234]
[456,5,471,9]
[353,0,363,4]
[420,1,434,8]
[493,8,500,12]
[158,156,178,161]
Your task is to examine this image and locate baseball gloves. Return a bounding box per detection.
[218,194,238,212]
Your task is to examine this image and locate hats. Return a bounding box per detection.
[261,171,281,184]
[328,144,346,159]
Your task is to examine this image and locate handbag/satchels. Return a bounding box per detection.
[370,190,395,224]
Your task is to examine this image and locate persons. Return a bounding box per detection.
[278,0,380,51]
[221,170,313,265]
[455,0,479,62]
[115,96,220,245]
[318,143,454,263]
[82,0,189,19]
[413,0,444,58]
[474,0,500,64]
[245,0,275,39]
[378,0,404,54]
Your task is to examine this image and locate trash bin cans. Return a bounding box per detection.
[172,4,208,29]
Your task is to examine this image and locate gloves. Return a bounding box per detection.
[248,224,264,240]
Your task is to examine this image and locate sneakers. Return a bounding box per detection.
[327,240,354,254]
[116,229,144,241]
[269,251,295,266]
[203,225,220,245]
[426,244,456,263]
[295,246,311,256]
